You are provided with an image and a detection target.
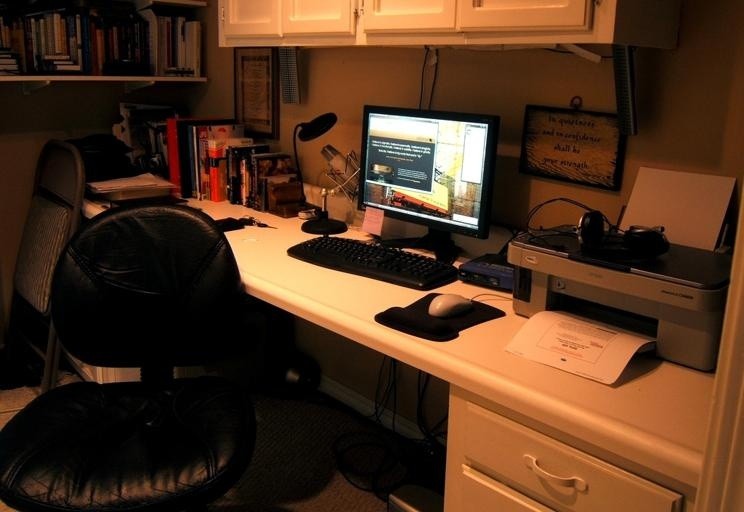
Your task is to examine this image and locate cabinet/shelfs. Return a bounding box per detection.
[443,380,695,511]
[360,0,682,54]
[0,0,208,99]
[219,2,360,47]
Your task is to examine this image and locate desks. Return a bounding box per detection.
[83,198,716,486]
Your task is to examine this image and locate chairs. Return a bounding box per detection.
[0,200,255,512]
[8,139,84,396]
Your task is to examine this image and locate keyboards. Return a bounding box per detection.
[287,236,459,291]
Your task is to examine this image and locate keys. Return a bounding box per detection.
[236,215,280,230]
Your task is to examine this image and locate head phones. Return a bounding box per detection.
[577,210,668,263]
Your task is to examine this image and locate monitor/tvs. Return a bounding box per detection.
[358,105,500,262]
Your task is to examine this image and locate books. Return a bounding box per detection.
[86,116,290,221]
[0,12,201,77]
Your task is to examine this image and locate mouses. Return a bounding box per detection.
[428,294,473,316]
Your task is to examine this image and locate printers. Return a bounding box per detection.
[508,224,726,372]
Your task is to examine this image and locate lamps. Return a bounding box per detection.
[292,111,337,212]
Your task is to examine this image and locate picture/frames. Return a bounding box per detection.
[519,104,628,192]
[231,47,280,142]
